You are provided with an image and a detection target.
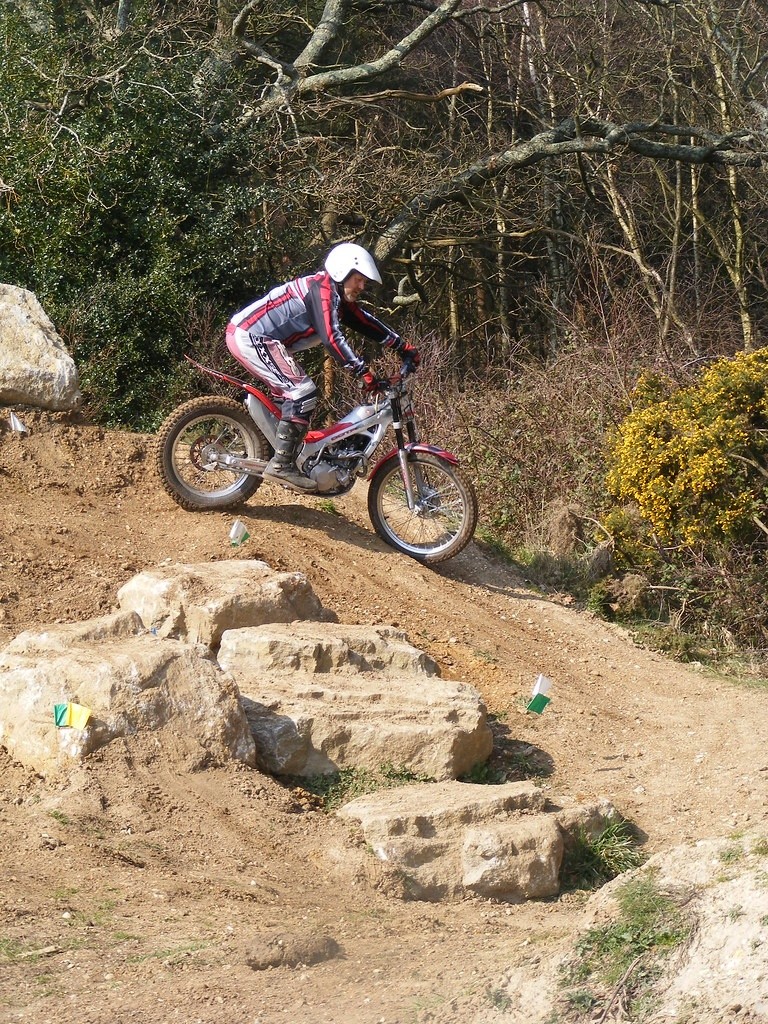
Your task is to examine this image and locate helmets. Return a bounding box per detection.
[325,243,383,301]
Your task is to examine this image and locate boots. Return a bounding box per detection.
[262,419,319,495]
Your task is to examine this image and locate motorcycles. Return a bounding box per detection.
[153,347,479,563]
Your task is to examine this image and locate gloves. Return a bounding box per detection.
[398,342,423,374]
[355,370,377,395]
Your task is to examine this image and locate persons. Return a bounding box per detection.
[227,242,421,493]
